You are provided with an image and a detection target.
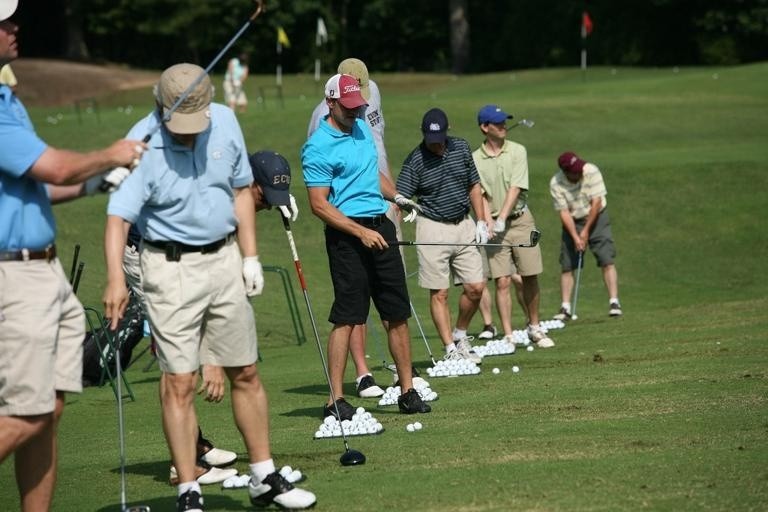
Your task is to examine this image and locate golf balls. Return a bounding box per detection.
[471,315,565,358]
[512,366,519,372]
[379,374,438,406]
[315,407,382,438]
[426,359,481,377]
[572,313,578,319]
[406,424,414,431]
[222,466,302,489]
[414,422,422,430]
[493,367,500,374]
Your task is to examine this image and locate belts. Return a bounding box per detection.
[143,229,236,254]
[492,211,524,220]
[418,208,470,224]
[127,239,138,252]
[0,242,56,263]
[348,212,386,227]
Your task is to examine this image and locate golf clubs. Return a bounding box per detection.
[387,230,541,247]
[115,318,151,512]
[410,301,438,366]
[561,250,582,318]
[507,118,534,131]
[96,1,265,194]
[276,205,366,464]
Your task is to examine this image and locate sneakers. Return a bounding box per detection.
[324,303,622,415]
[160,425,317,512]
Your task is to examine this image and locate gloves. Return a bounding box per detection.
[279,193,298,222]
[239,255,265,299]
[396,194,423,222]
[85,159,140,195]
[492,219,505,233]
[476,221,488,247]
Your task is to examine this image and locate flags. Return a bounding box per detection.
[583,12,592,35]
[279,26,289,46]
[316,18,328,44]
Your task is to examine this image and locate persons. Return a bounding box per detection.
[474,249,529,342]
[2,64,17,93]
[549,149,624,320]
[0,0,150,511]
[104,63,320,510]
[469,102,553,348]
[221,55,252,111]
[307,55,423,400]
[298,74,430,421]
[121,150,299,486]
[396,108,488,368]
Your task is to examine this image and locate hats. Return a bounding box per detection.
[477,104,513,125]
[337,58,372,103]
[249,150,292,206]
[324,74,370,110]
[157,63,213,135]
[559,152,586,173]
[422,108,448,144]
[0,0,18,22]
